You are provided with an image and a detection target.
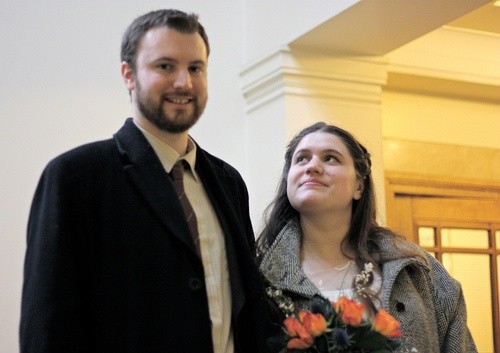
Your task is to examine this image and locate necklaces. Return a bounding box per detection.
[303,240,355,272]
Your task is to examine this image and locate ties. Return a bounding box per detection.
[168,162,201,261]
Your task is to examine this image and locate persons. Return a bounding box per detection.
[15,7,294,353]
[255,121,484,352]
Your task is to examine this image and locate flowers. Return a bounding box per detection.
[265,263,402,353]
